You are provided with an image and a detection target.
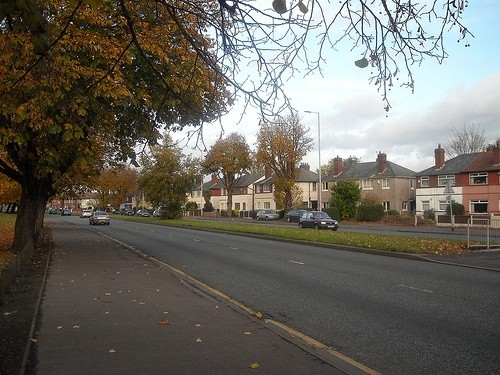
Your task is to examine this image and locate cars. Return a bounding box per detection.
[256,209,280,221]
[49,208,58,214]
[153,210,162,218]
[61,209,71,216]
[137,209,150,217]
[286,209,306,223]
[120,208,134,216]
[80,210,92,218]
[298,212,339,231]
[89,212,110,225]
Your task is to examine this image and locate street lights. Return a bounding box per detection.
[304,110,323,212]
[191,147,203,217]
